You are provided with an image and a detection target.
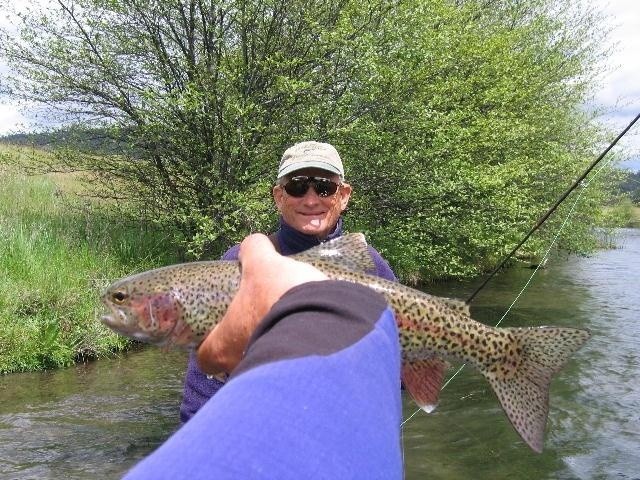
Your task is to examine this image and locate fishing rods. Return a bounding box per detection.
[401,112,640,390]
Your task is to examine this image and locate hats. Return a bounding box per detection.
[278,140,345,178]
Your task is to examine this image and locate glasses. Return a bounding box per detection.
[282,176,340,195]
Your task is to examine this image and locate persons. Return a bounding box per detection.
[179,140,402,421]
[123,234,408,480]
